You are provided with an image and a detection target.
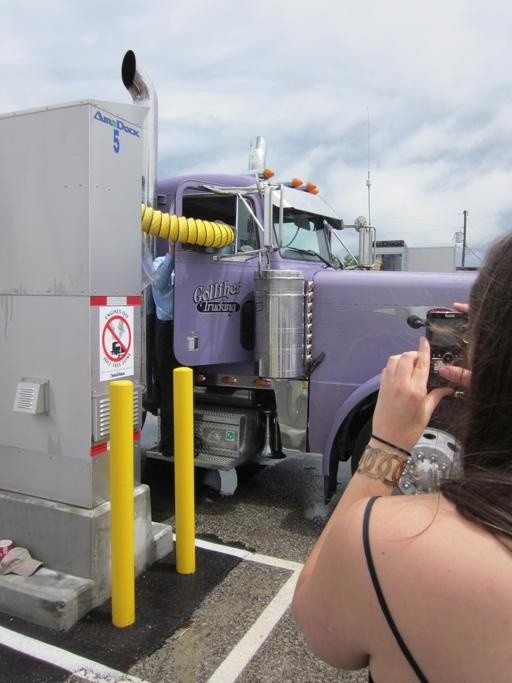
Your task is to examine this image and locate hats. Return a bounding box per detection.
[1,546,44,577]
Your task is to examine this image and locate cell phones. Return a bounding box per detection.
[423,308,470,393]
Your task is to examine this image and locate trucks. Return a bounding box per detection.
[122,49,494,505]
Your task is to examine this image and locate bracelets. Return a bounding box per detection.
[357,444,407,487]
[370,433,411,456]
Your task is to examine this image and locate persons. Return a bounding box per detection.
[151,241,206,457]
[290,225,511,682]
